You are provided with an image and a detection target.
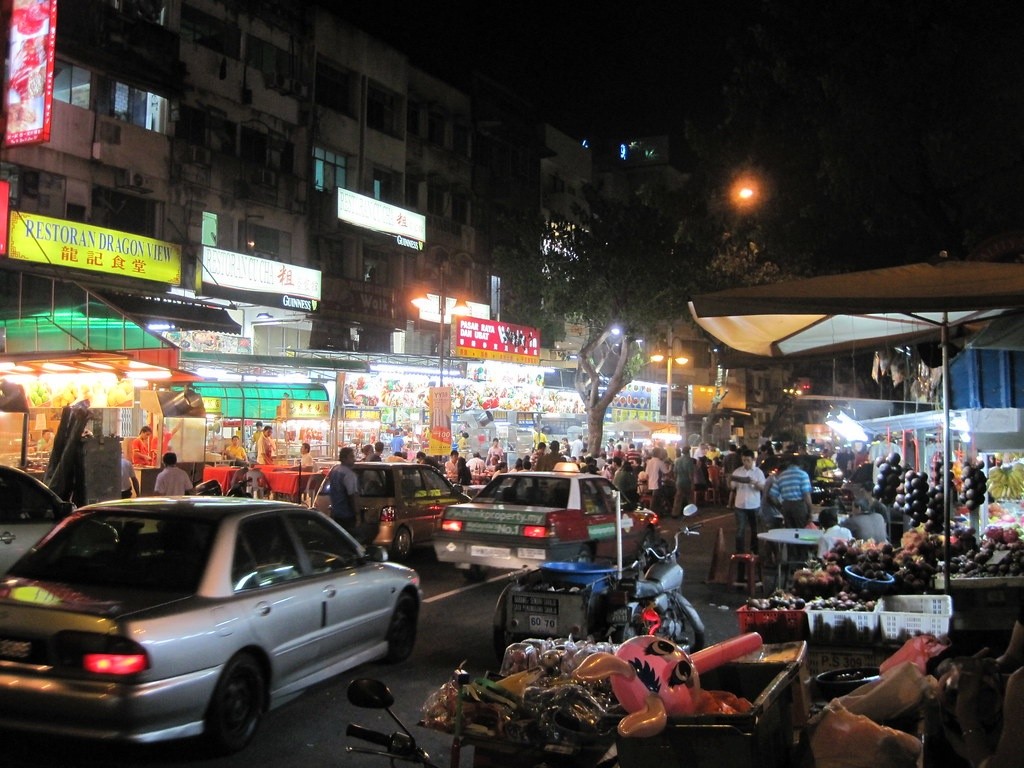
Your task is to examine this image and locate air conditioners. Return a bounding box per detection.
[190,144,212,169]
[292,79,305,97]
[254,169,277,189]
[122,170,154,195]
[267,70,292,92]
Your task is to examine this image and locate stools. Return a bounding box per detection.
[704,488,721,505]
[730,553,765,596]
[641,496,652,510]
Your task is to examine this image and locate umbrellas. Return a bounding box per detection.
[687,251,1024,595]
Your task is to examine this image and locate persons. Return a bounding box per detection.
[130,419,184,466]
[122,447,139,500]
[154,452,194,497]
[248,421,274,487]
[754,440,890,569]
[727,449,766,556]
[360,427,748,519]
[300,443,313,467]
[328,448,359,539]
[222,436,250,472]
[37,427,56,452]
[989,455,995,503]
[955,610,1024,768]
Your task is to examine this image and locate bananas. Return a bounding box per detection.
[986,462,1024,500]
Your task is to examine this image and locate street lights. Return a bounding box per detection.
[666,336,689,424]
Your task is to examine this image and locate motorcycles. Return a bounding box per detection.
[491,503,706,669]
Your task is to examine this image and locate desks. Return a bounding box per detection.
[263,470,326,503]
[812,513,850,522]
[472,474,492,485]
[768,528,825,589]
[758,532,824,591]
[204,466,244,493]
[254,464,300,489]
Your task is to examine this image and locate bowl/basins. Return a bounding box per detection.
[539,561,615,592]
[845,566,894,595]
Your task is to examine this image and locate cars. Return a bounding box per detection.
[0,465,118,576]
[314,462,473,565]
[1,496,425,768]
[433,470,658,570]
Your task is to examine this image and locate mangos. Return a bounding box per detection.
[30,381,132,409]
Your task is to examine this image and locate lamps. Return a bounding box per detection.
[824,409,868,442]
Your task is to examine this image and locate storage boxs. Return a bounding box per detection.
[736,604,806,644]
[876,594,954,645]
[731,640,813,729]
[809,652,885,676]
[936,571,1024,589]
[805,600,880,643]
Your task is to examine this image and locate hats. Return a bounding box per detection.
[580,448,588,453]
[682,445,691,453]
[877,433,884,439]
[598,449,607,456]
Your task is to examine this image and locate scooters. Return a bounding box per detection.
[344,678,437,768]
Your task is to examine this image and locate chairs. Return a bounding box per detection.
[242,471,265,498]
[525,486,539,505]
[304,473,326,507]
[500,486,518,502]
[214,459,236,468]
[160,522,202,552]
[363,480,380,495]
[226,469,247,496]
[402,479,417,497]
[592,493,608,513]
